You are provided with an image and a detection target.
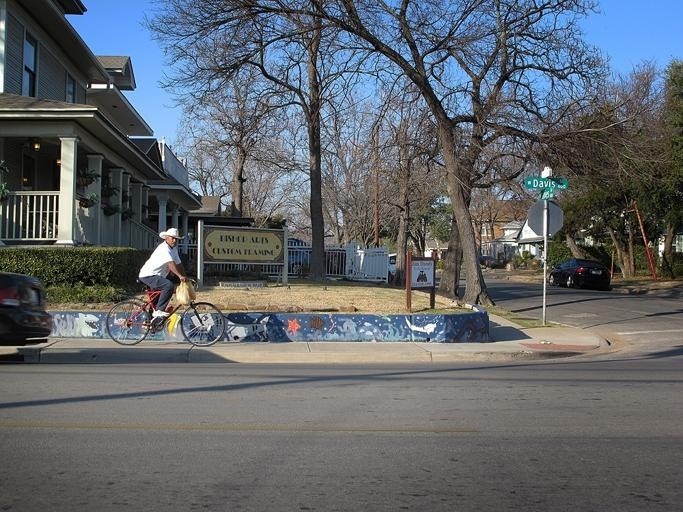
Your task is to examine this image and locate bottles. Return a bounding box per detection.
[159,228,186,240]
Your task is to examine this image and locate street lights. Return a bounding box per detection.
[176,281,196,305]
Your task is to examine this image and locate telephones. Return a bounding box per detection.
[523,177,569,191]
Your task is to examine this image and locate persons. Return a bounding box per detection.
[138,228,188,330]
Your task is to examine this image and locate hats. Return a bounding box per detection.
[547,258,611,291]
[0,270,50,346]
[287,239,312,276]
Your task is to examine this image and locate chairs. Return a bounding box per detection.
[151,310,170,317]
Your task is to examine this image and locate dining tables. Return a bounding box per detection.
[78,193,134,223]
